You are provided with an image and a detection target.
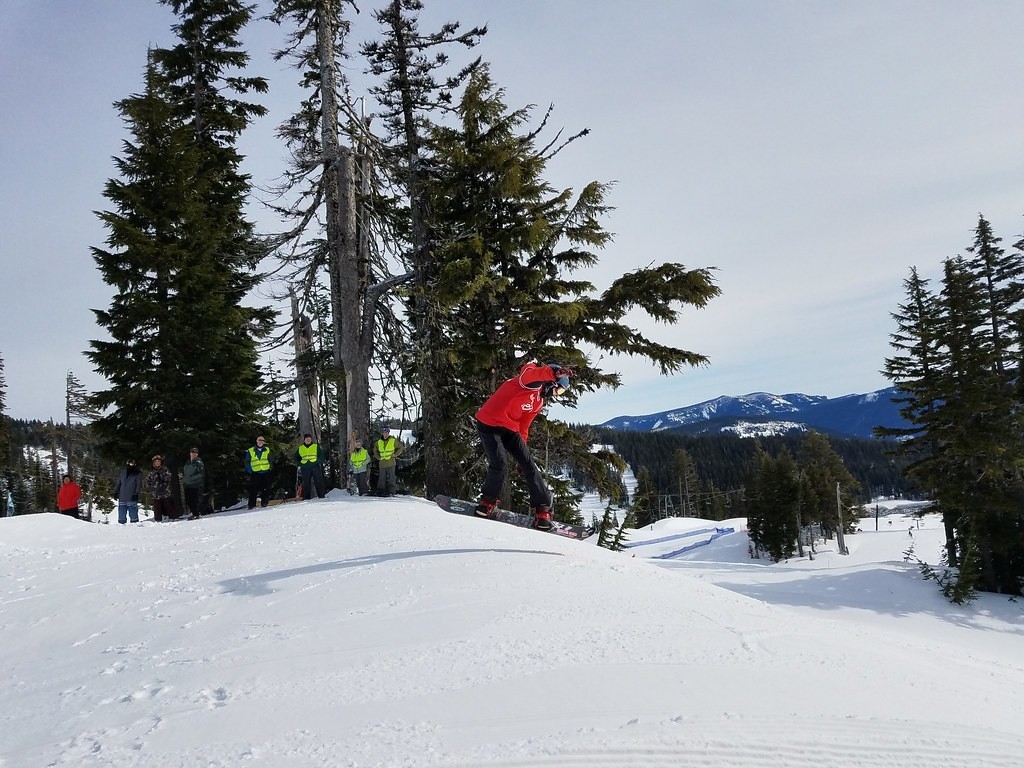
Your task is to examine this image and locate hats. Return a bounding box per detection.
[304,434,311,440]
[382,428,390,432]
[354,438,363,447]
[152,455,162,461]
[190,448,198,451]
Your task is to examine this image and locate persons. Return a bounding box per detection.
[475,359,577,531]
[245,436,273,508]
[373,428,404,497]
[113,448,204,524]
[349,438,372,496]
[56,474,82,518]
[294,434,326,501]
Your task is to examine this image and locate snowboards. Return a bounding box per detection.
[434,493,597,541]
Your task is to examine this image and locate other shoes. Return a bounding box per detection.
[188,515,199,520]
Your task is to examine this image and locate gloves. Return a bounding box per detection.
[131,495,138,501]
[555,368,576,377]
[114,492,118,501]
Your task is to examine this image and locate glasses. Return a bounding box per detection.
[553,383,565,394]
[259,440,264,442]
[192,451,199,453]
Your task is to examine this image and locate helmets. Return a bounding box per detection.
[127,458,137,467]
[542,364,569,399]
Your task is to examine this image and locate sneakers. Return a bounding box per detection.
[475,500,503,520]
[533,510,551,530]
